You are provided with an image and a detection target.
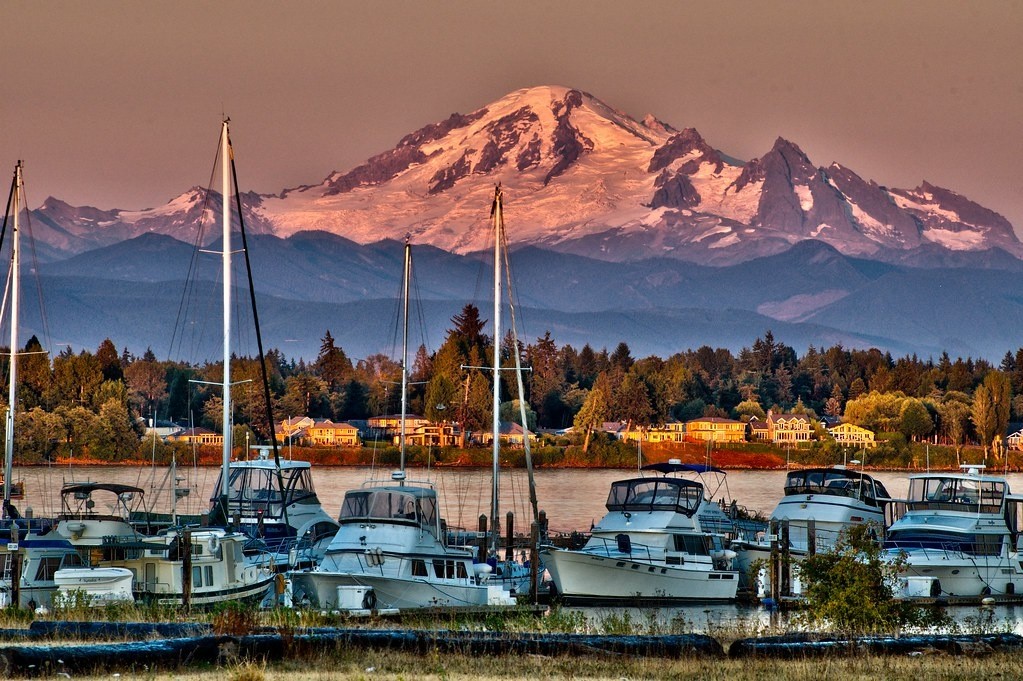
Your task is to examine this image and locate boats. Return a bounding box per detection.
[1,115,552,614]
[855,458,1023,594]
[731,467,893,598]
[538,459,743,607]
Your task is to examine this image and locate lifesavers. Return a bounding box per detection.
[364,590,376,609]
[208,533,221,554]
